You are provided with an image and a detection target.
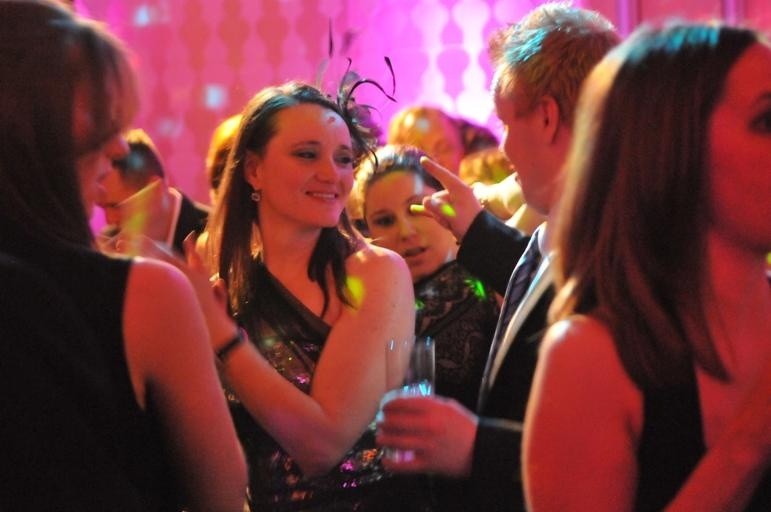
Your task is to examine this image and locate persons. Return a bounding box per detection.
[0,1,771,511]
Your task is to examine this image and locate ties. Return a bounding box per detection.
[478,228,542,414]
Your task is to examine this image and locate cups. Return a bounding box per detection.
[383,336,436,464]
